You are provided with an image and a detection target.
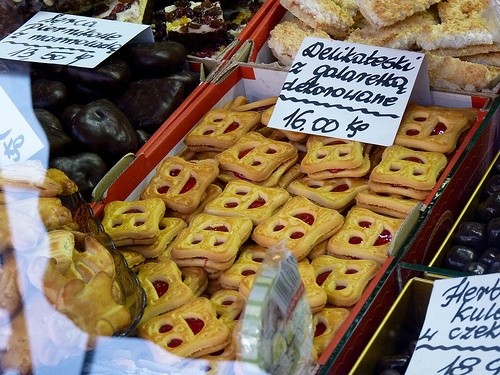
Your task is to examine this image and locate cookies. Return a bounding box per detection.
[0,94,479,375]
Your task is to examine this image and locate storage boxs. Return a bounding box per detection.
[78,0,500,375]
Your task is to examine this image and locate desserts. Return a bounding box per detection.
[0,0,266,208]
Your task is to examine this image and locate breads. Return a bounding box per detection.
[257,0,500,93]
